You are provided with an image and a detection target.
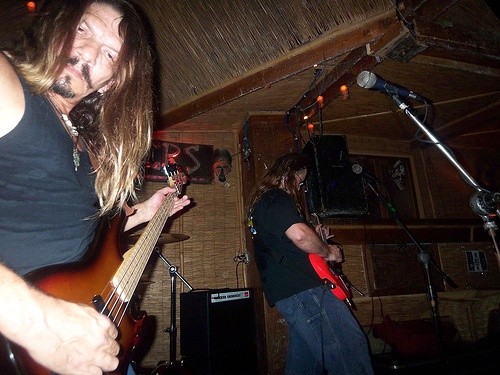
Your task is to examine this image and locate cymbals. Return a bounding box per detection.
[123,232,191,246]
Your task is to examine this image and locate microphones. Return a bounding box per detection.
[352,164,379,184]
[357,71,425,104]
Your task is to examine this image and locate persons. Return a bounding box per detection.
[0,0,191,375]
[245,151,376,375]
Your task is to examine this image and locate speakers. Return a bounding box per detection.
[179,287,260,375]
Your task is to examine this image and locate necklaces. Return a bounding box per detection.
[46,92,81,173]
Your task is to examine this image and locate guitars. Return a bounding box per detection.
[308,210,351,301]
[0,159,188,374]
